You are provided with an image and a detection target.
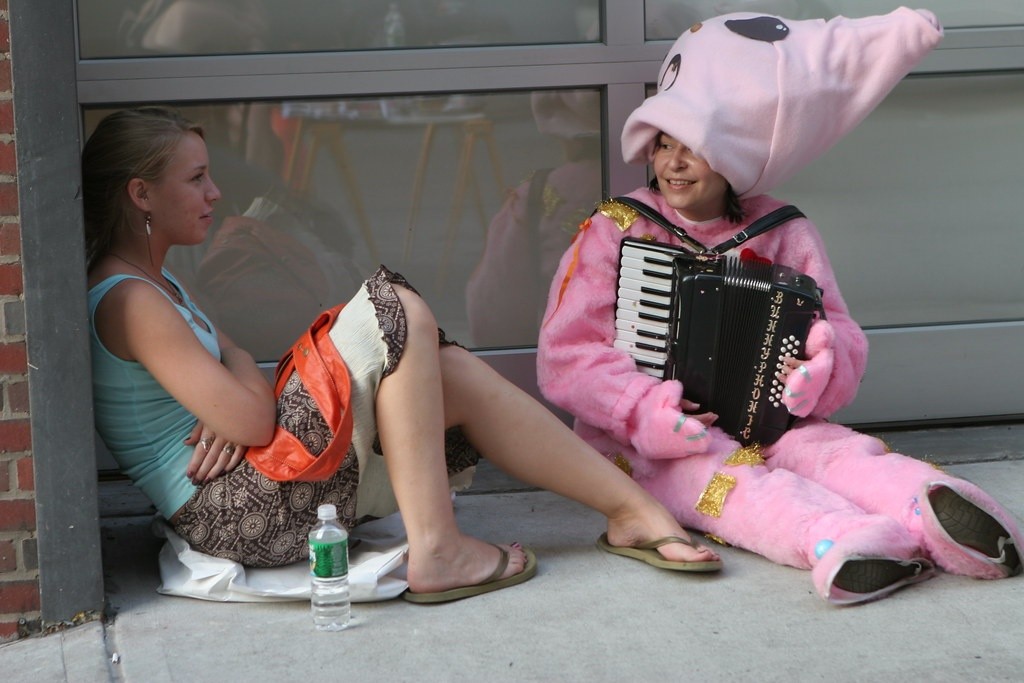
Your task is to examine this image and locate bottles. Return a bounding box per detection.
[309,504,352,633]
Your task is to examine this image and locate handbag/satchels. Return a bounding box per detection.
[246,303,354,482]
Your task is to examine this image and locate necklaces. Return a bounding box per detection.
[110,248,184,309]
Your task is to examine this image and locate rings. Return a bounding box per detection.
[200,435,213,453]
[224,447,235,454]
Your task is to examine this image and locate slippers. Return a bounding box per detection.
[598,530,724,571]
[403,543,537,603]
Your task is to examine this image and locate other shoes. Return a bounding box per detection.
[930,486,1022,576]
[832,559,929,595]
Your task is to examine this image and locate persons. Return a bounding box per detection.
[78,108,726,603]
[535,130,1020,596]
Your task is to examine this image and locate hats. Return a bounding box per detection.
[623,5,944,191]
